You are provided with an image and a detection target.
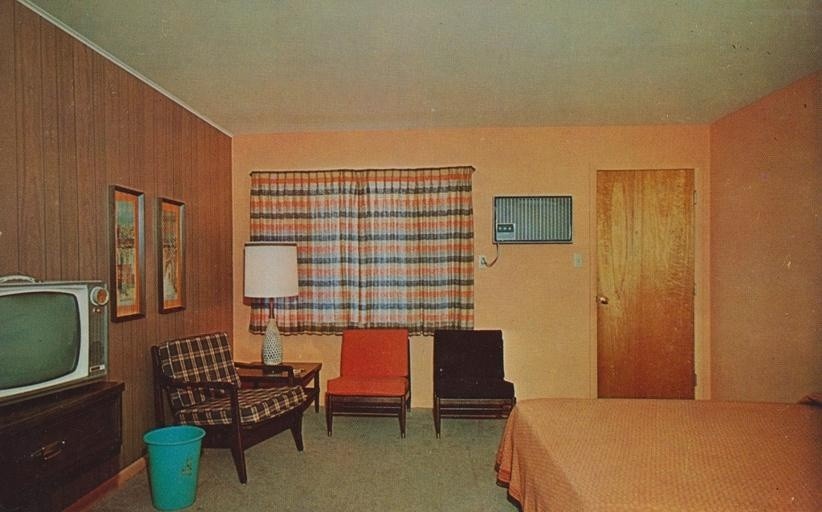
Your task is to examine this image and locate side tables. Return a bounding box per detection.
[237,362,322,413]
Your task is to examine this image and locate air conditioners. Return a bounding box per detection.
[485,196,573,271]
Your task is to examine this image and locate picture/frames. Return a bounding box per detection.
[154,196,188,314]
[109,184,147,323]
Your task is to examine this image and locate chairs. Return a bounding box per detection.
[151,332,319,486]
[324,327,412,440]
[433,328,517,438]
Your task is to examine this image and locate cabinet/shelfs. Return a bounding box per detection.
[0,393,124,512]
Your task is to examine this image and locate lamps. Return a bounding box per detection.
[243,240,299,363]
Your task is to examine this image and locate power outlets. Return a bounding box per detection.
[479,255,485,270]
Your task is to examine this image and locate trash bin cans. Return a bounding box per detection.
[143,426,206,511]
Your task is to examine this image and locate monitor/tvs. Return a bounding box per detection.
[0,283,109,398]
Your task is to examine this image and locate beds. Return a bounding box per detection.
[495,397,822,512]
